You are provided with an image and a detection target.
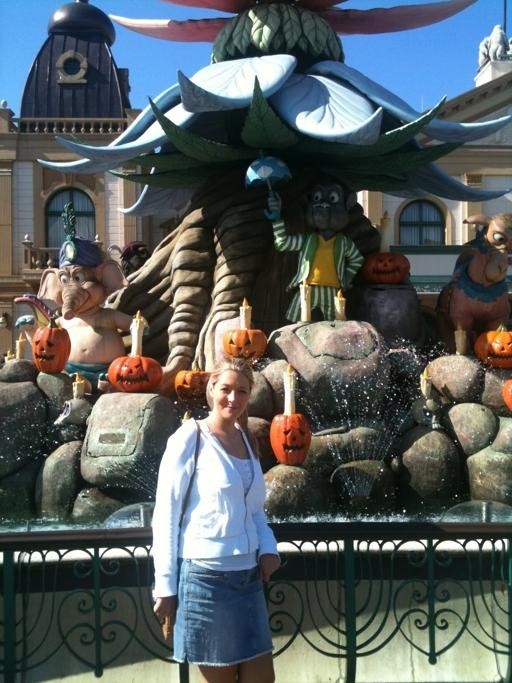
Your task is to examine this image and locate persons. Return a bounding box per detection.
[151,357,281,683]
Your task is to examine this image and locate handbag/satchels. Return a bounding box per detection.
[152,590,173,640]
